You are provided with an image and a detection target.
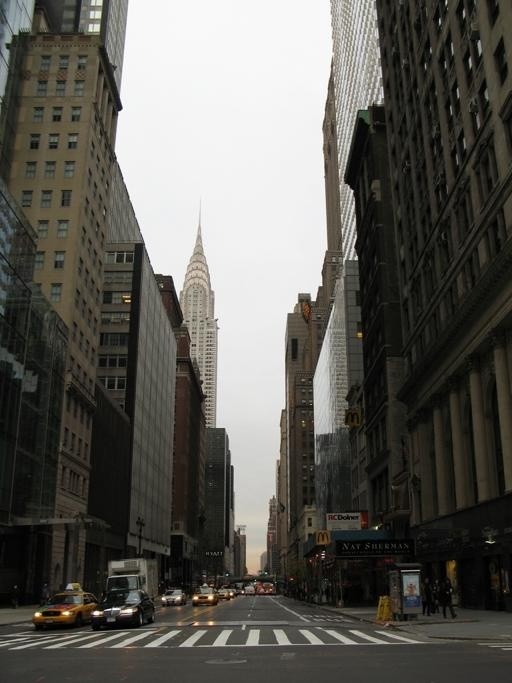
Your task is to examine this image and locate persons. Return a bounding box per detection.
[40,583,49,606]
[420,578,457,618]
[11,585,18,609]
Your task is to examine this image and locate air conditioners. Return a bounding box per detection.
[468,23,479,41]
[439,232,447,245]
[469,97,478,113]
[402,160,410,173]
[432,125,440,139]
[436,173,446,186]
[401,58,409,70]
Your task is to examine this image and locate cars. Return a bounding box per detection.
[32,582,98,629]
[90,586,155,631]
[192,580,277,606]
[161,588,187,607]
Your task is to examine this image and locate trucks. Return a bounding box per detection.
[106,556,160,608]
